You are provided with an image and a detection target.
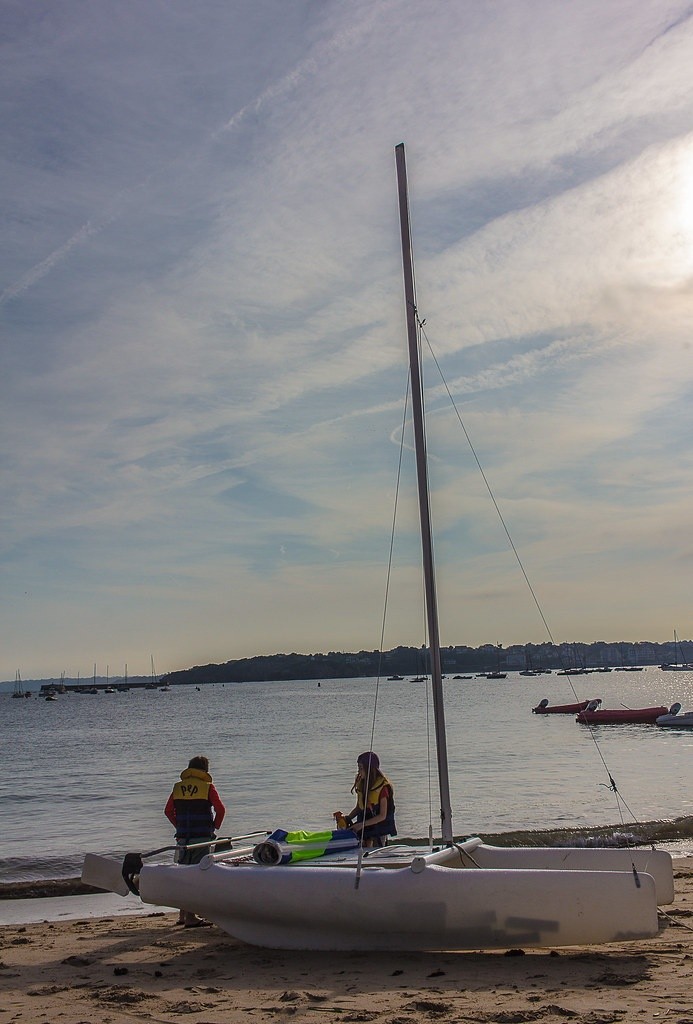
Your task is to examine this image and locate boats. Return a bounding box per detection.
[576,700,669,725]
[531,698,602,714]
[655,702,693,726]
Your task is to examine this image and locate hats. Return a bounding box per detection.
[357,751,380,769]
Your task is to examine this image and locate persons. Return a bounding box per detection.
[164,756,226,927]
[334,751,397,847]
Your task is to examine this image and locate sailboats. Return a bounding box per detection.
[80,142,673,951]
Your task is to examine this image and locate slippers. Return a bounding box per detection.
[174,918,185,925]
[185,919,213,928]
